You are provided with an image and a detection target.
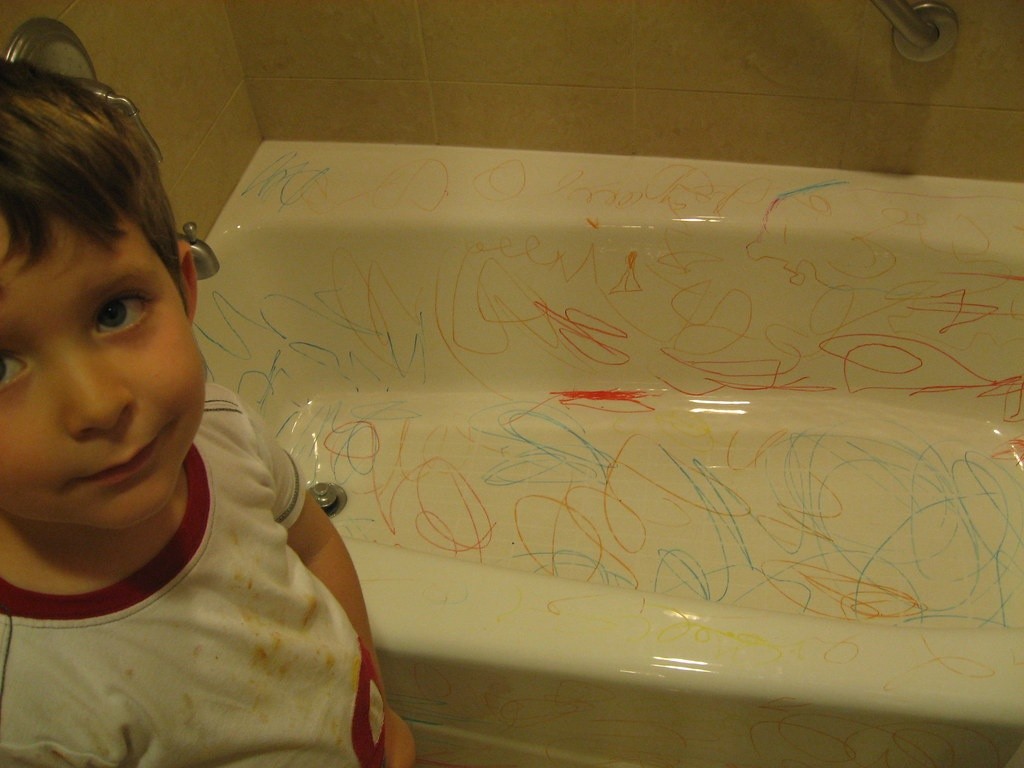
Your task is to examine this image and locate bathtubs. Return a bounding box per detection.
[182,142,1024,767]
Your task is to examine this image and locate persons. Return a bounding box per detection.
[0,61,415,768]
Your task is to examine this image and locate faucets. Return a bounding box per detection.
[175,221,221,279]
